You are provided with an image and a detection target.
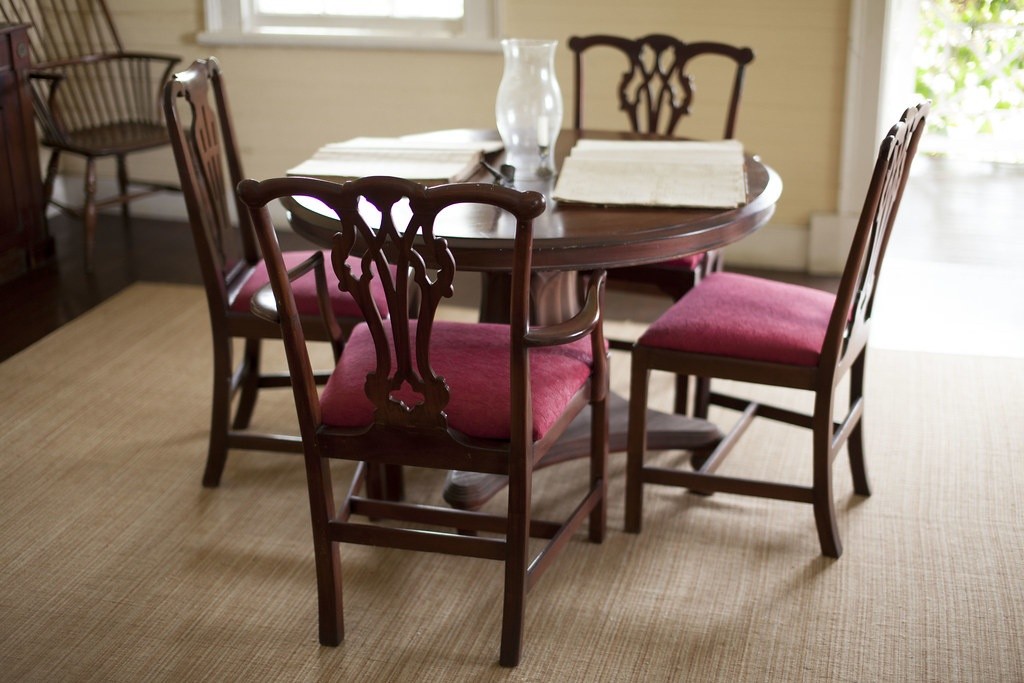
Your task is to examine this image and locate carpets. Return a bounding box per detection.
[0,282,1023,683]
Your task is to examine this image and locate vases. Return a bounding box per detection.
[496,38,563,190]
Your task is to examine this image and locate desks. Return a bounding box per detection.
[279,123,783,538]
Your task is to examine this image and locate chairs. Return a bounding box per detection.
[565,31,756,414]
[235,176,606,666]
[168,56,416,488]
[0,1,193,298]
[621,101,925,557]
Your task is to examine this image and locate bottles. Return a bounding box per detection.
[495,38,565,182]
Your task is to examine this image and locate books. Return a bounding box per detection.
[552,139,747,210]
[287,138,503,187]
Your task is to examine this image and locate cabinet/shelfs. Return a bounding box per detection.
[0,20,58,285]
